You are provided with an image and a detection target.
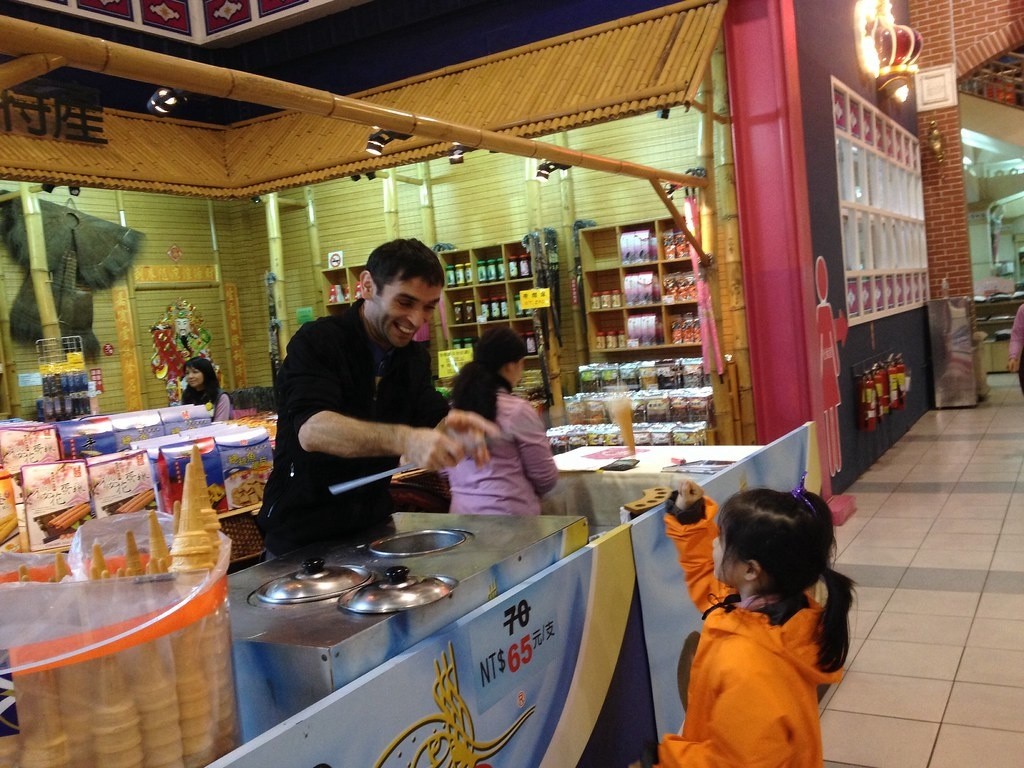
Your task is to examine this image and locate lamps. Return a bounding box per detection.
[351,174,361,182]
[536,162,558,181]
[251,196,262,205]
[365,129,393,156]
[146,88,190,118]
[69,186,81,197]
[366,171,376,181]
[447,144,467,164]
[41,183,56,194]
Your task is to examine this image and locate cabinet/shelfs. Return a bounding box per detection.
[577,215,706,365]
[321,261,369,316]
[972,297,1024,373]
[925,296,989,412]
[434,235,568,429]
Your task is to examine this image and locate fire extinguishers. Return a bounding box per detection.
[858,352,907,432]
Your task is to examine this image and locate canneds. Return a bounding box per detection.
[445,253,537,356]
[591,290,626,349]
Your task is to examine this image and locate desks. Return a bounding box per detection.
[541,445,768,529]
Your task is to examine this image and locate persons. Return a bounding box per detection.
[1008,304,1024,396]
[182,356,233,422]
[629,471,853,768]
[446,327,558,515]
[256,239,501,562]
